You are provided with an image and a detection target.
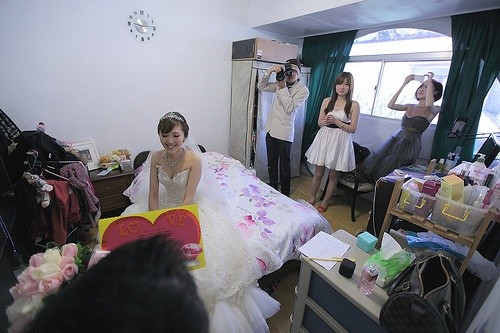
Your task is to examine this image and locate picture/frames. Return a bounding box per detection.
[71,142,102,171]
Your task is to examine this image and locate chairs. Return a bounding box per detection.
[304,130,375,222]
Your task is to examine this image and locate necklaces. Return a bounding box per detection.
[164,148,186,167]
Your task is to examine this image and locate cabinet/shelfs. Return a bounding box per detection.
[377,160,496,281]
[288,229,397,333]
[230,58,313,182]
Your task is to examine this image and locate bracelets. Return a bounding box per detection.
[339,123,345,129]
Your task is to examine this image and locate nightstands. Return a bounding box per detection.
[88,167,137,218]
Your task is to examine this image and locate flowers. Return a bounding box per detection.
[6,240,112,333]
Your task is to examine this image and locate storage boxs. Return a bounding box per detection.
[440,175,464,203]
[232,38,299,63]
[433,185,495,237]
[399,177,434,217]
[357,231,378,253]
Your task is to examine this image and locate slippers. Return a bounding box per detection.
[317,203,330,212]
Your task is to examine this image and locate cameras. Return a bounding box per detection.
[275,62,293,81]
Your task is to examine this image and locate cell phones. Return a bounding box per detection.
[413,75,424,81]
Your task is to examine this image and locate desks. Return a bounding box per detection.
[360,162,500,238]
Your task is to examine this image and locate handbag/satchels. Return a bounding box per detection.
[379,249,466,333]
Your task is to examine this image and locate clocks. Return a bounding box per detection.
[128,9,157,42]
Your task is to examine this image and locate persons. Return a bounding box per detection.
[304,72,361,213]
[19,232,209,333]
[363,72,443,187]
[88,110,283,333]
[257,59,310,197]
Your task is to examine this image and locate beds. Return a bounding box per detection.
[134,144,333,291]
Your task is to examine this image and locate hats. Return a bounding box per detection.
[285,58,302,77]
